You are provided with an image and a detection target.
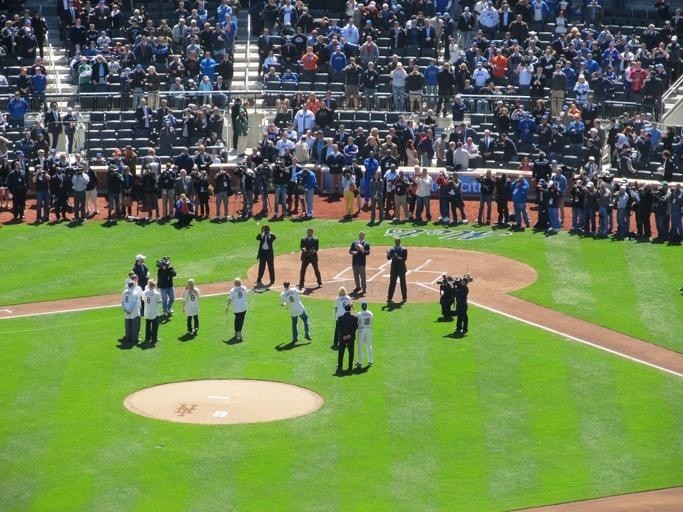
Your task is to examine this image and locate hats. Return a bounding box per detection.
[136,254,146,261]
[362,301,367,308]
[461,278,468,283]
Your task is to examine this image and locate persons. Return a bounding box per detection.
[331,286,354,347]
[435,276,454,322]
[223,278,248,341]
[181,279,201,336]
[353,298,374,368]
[345,230,371,295]
[251,224,278,287]
[0,0,682,250]
[278,280,312,343]
[295,227,324,291]
[333,305,358,375]
[385,238,409,304]
[447,278,468,333]
[116,254,179,348]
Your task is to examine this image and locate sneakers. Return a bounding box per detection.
[355,362,362,367]
[318,283,322,288]
[169,307,173,314]
[305,335,311,341]
[368,363,373,366]
[193,328,198,335]
